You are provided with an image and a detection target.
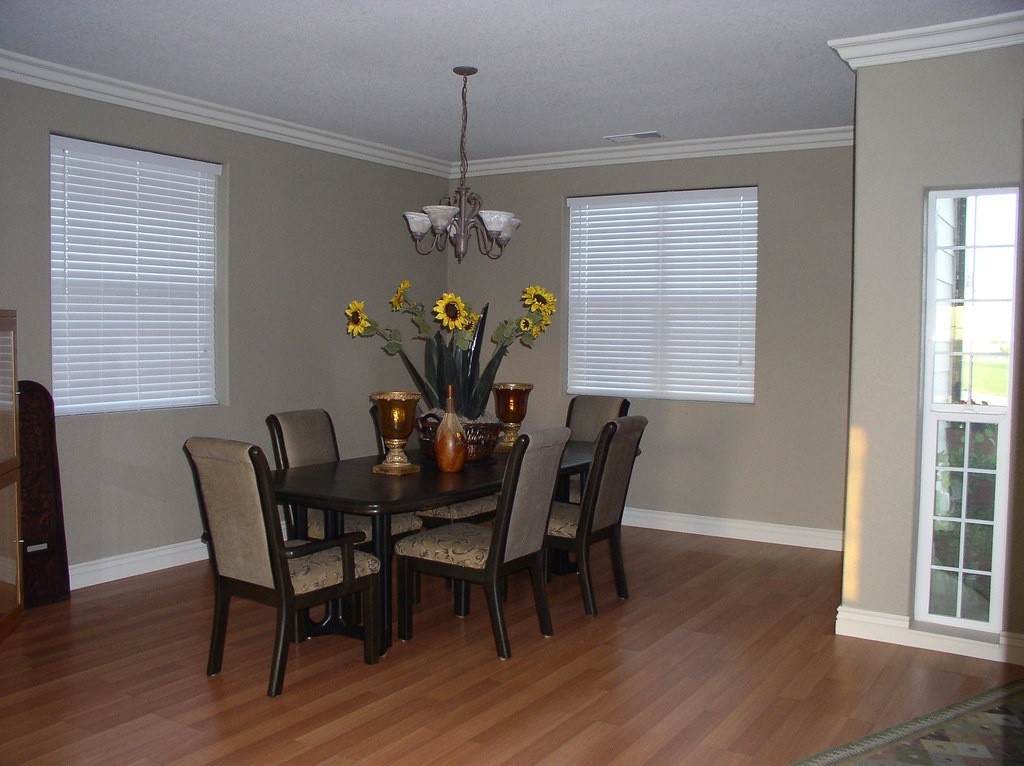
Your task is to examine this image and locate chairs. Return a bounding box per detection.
[543,414,649,617]
[264,408,424,629]
[184,436,380,697]
[552,396,631,578]
[369,404,501,592]
[394,426,572,660]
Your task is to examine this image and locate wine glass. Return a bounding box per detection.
[492,382,534,452]
[371,390,421,474]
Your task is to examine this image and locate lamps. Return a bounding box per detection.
[404,66,521,263]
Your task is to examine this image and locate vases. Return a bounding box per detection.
[491,382,534,454]
[368,391,422,475]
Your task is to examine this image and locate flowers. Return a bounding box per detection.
[345,281,556,419]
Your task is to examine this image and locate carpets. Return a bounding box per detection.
[791,678,1024,766]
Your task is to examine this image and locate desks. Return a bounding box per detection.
[271,441,642,664]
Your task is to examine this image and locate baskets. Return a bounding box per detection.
[415,413,503,460]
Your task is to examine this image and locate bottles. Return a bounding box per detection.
[434,384,467,473]
